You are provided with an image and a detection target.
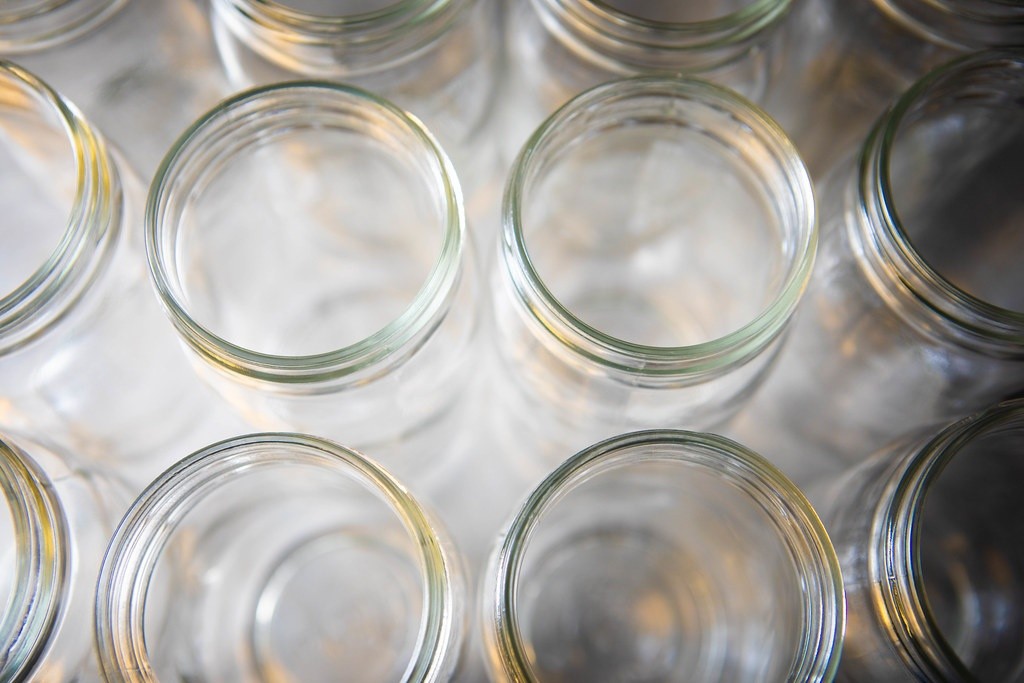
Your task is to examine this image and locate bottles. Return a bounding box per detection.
[1,0,1023,681]
